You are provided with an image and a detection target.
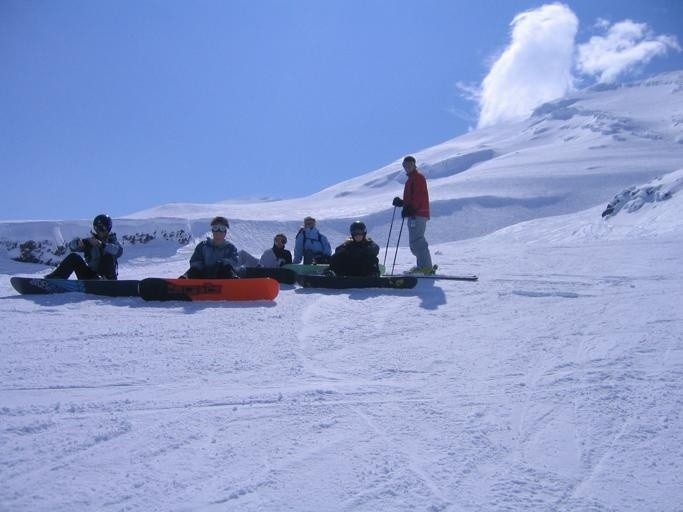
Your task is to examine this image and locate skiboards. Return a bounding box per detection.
[384,273,478,280]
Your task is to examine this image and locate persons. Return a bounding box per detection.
[292,217,331,268]
[331,221,381,277]
[176,216,239,281]
[255,235,292,274]
[43,215,123,283]
[392,156,435,276]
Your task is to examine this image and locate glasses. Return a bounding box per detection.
[212,224,226,232]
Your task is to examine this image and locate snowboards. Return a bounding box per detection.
[237,264,417,288]
[11,278,280,300]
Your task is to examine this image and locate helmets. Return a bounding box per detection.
[350,221,367,236]
[403,157,415,166]
[93,215,112,233]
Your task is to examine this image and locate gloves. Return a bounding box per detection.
[393,197,403,207]
[402,207,415,217]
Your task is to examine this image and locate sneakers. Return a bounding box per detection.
[404,266,434,274]
